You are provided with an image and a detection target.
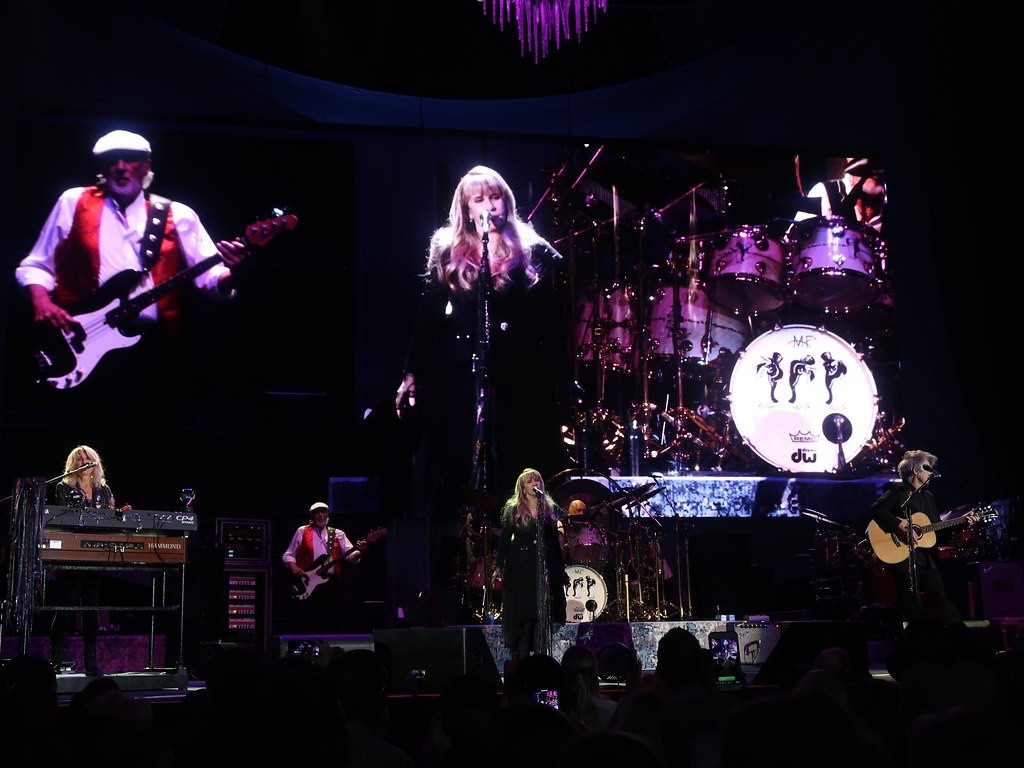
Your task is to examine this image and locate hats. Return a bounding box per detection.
[309,502,329,511]
[92,129,152,156]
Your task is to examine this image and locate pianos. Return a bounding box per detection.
[22,509,201,676]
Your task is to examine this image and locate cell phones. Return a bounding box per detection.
[708,631,744,691]
[288,640,320,661]
[536,690,559,711]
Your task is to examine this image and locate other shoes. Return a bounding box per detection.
[52,662,78,674]
[86,668,103,676]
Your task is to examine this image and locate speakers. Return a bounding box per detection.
[973,560,1024,622]
[727,622,783,664]
[754,621,869,685]
[373,627,505,696]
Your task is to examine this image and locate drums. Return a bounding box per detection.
[562,564,610,624]
[468,556,505,594]
[725,321,882,475]
[565,525,608,563]
[787,217,884,315]
[701,222,788,315]
[575,281,671,389]
[640,280,748,384]
[626,539,674,589]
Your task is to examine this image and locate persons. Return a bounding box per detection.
[57,444,115,509]
[0,628,1024,768]
[783,157,886,269]
[282,501,367,633]
[395,165,575,569]
[17,129,247,335]
[492,468,570,657]
[873,450,976,629]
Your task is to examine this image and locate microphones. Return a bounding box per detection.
[479,210,492,220]
[648,475,663,478]
[83,462,98,466]
[923,464,942,478]
[833,416,847,426]
[532,487,543,495]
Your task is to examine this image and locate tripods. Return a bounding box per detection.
[603,507,689,624]
[561,174,756,475]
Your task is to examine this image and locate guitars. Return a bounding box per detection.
[288,525,389,602]
[865,504,1000,566]
[21,204,300,400]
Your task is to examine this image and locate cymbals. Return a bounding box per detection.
[800,508,841,525]
[590,483,656,510]
[626,486,666,509]
